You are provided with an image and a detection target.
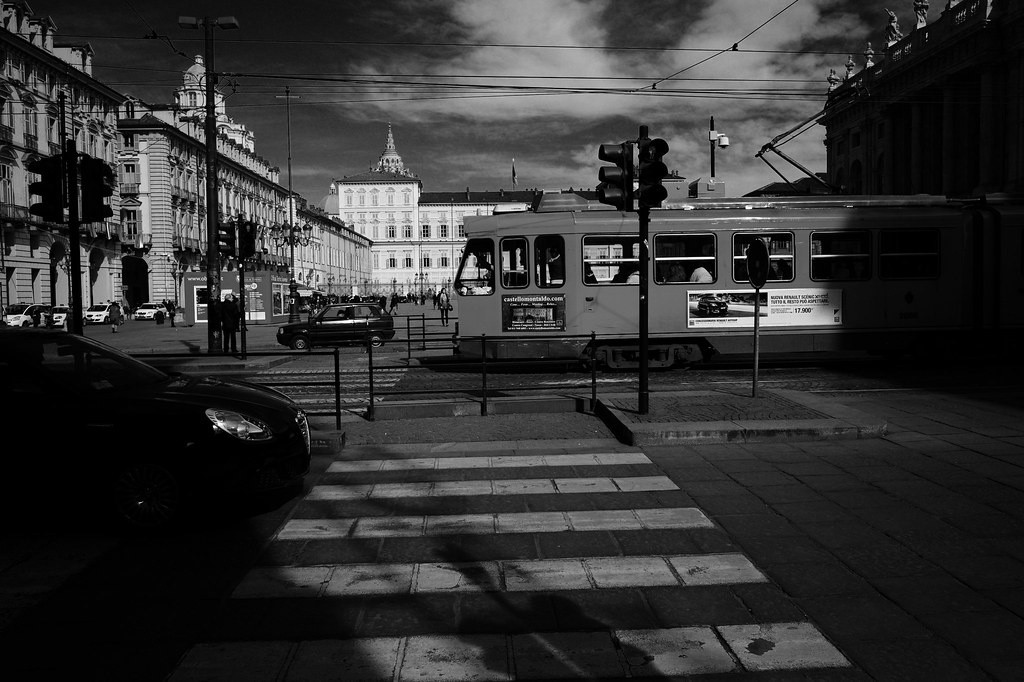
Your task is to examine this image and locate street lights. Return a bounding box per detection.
[415,272,428,299]
[170,261,183,306]
[64,249,71,310]
[270,220,313,323]
[178,16,241,356]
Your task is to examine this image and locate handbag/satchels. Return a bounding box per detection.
[448,303,453,311]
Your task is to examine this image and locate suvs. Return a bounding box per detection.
[276,301,396,351]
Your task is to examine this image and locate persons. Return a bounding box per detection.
[274,292,437,313]
[2,300,176,328]
[220,294,240,352]
[437,288,452,327]
[585,248,859,283]
[19,344,47,387]
[109,301,120,333]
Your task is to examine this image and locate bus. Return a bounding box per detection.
[452,80,1023,373]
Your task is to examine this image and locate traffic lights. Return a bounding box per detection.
[79,156,113,223]
[239,221,256,256]
[219,221,235,258]
[27,153,64,224]
[598,139,628,212]
[638,136,669,209]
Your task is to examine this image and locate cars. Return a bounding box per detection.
[134,302,170,321]
[0,319,313,539]
[2,301,89,329]
[84,303,125,325]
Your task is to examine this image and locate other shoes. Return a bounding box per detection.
[232,349,239,352]
[446,325,449,327]
[224,350,229,352]
[112,328,115,333]
[395,312,397,315]
